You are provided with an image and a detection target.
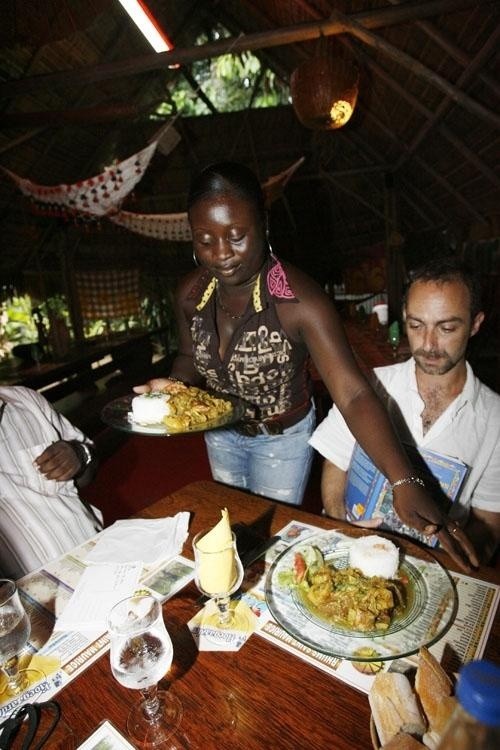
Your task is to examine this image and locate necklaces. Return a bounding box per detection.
[216,299,245,320]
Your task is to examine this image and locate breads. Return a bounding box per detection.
[367,645,459,750]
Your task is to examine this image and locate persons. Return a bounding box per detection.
[307,254,500,565]
[33,313,48,361]
[0,385,105,584]
[131,160,485,576]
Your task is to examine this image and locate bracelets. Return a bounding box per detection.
[391,475,426,489]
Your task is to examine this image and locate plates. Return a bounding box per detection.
[261,530,461,666]
[104,388,244,435]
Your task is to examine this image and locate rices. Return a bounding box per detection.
[131,394,171,427]
[349,535,401,581]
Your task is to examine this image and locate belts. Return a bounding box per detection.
[234,396,312,437]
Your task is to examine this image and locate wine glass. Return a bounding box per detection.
[0,577,49,716]
[106,596,182,745]
[191,526,244,645]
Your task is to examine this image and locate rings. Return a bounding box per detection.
[449,519,460,535]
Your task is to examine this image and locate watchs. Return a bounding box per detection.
[80,443,95,466]
